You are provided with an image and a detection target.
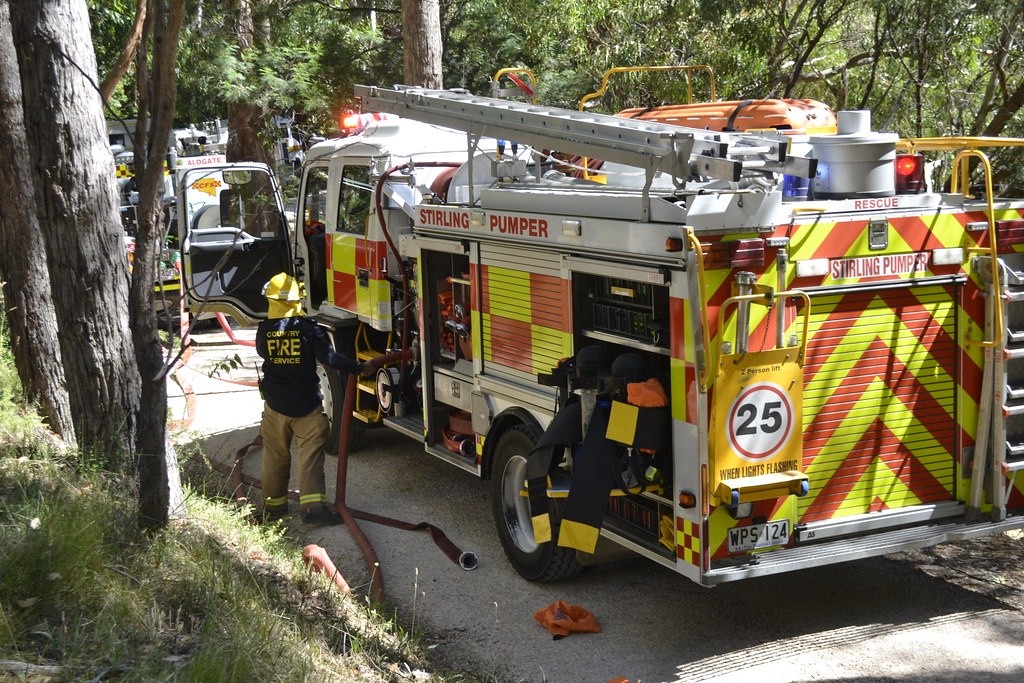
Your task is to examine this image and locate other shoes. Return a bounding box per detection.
[299,506,344,524]
[263,509,286,521]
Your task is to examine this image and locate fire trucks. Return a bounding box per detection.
[105,66,1024,588]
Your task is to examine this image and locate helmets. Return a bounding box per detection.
[264,272,300,300]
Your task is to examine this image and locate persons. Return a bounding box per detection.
[255,272,341,527]
